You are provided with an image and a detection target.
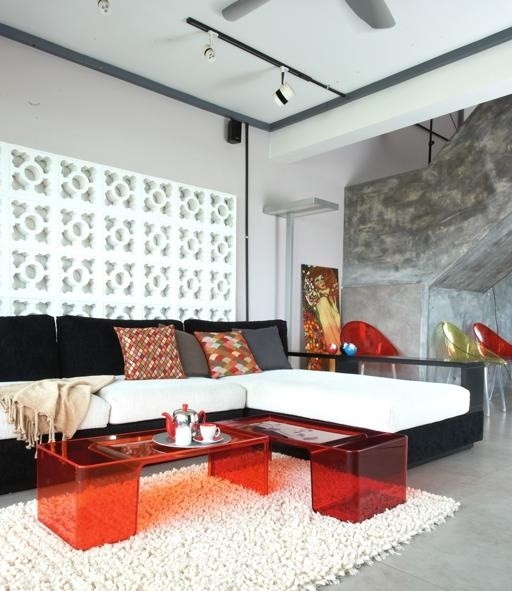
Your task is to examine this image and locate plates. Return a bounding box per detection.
[193,436,223,444]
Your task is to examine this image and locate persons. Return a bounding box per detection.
[312,274,340,348]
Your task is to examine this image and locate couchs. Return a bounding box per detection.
[0,351,484,495]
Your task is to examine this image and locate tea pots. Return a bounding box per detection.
[162,404,207,440]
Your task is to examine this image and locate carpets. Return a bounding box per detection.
[0,450,460,591]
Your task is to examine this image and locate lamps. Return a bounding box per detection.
[199,31,219,63]
[272,64,293,109]
[96,0,110,14]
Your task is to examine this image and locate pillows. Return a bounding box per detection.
[0,315,293,380]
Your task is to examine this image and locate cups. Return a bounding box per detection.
[175,426,192,446]
[199,424,220,440]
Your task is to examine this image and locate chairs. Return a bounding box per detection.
[442,322,507,419]
[472,322,512,400]
[340,321,398,379]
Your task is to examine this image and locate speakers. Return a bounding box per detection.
[228,119,242,143]
